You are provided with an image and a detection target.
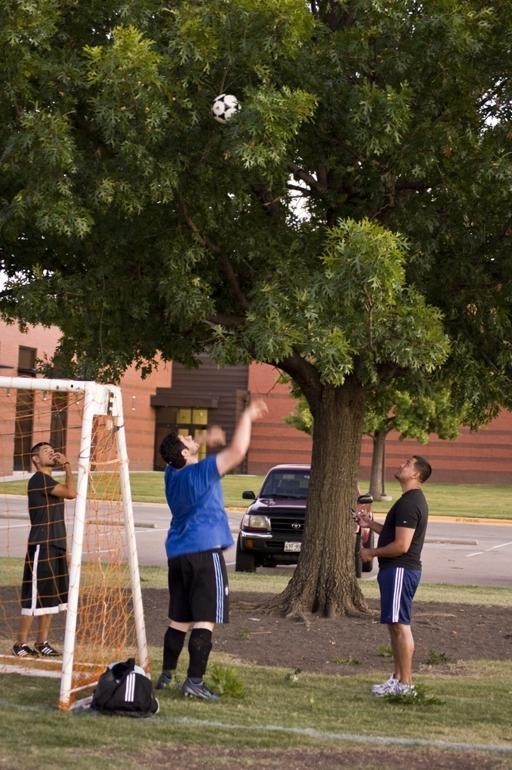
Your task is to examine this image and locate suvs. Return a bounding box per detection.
[235,463,374,574]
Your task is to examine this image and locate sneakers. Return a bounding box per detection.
[371,673,398,691]
[384,684,418,698]
[11,641,39,659]
[157,671,181,689]
[181,677,217,700]
[33,640,59,657]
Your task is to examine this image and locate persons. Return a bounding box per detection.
[350,454,433,699]
[12,441,77,659]
[157,399,270,700]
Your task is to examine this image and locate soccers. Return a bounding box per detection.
[209,93,243,125]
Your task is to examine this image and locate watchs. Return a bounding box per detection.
[62,461,70,468]
